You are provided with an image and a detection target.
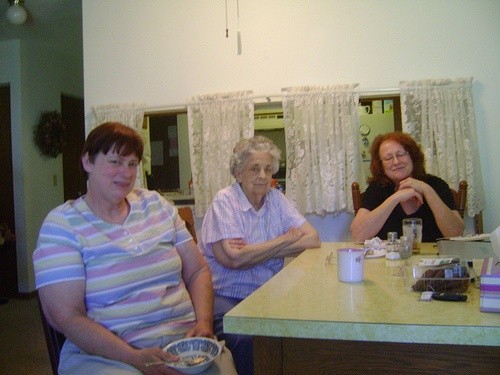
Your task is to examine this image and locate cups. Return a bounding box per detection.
[338,248,363,281]
[403,217,423,254]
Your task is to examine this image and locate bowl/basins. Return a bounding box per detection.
[161,336,221,375]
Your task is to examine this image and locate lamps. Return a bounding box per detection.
[7,0,28,24]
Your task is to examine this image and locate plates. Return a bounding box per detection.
[366,249,387,258]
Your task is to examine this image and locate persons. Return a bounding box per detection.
[197,136,320,375]
[31,121,237,375]
[349,132,465,245]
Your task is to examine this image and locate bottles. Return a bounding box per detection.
[385,233,399,259]
[398,236,411,259]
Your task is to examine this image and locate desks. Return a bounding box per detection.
[222,241,500,375]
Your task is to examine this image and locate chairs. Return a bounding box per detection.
[352,180,467,218]
[36,208,199,374]
